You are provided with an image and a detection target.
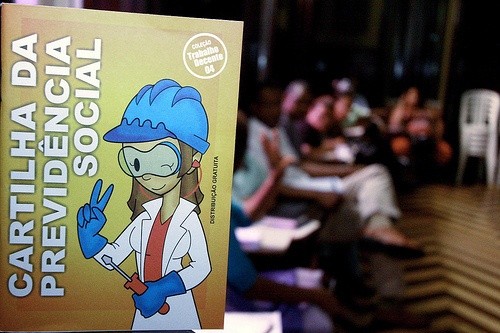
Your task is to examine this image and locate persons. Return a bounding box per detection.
[225,80,452,333]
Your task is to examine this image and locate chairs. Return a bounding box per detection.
[456,88,500,185]
